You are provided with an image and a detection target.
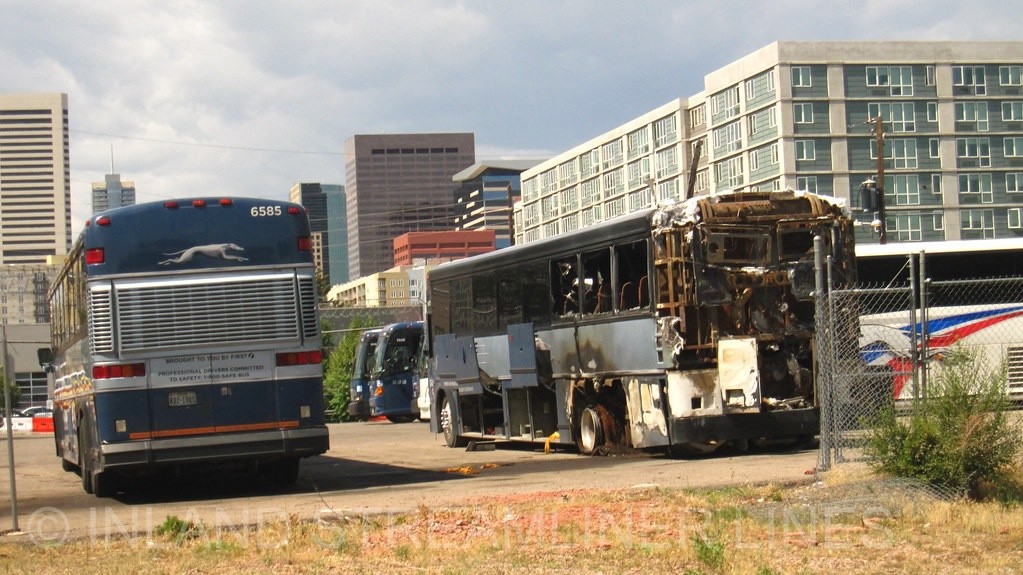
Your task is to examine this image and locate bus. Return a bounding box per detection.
[411,332,431,422]
[46,194,330,500]
[347,329,382,418]
[423,186,858,457]
[850,235,1023,417]
[368,321,426,424]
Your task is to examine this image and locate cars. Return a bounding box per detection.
[14,405,55,419]
